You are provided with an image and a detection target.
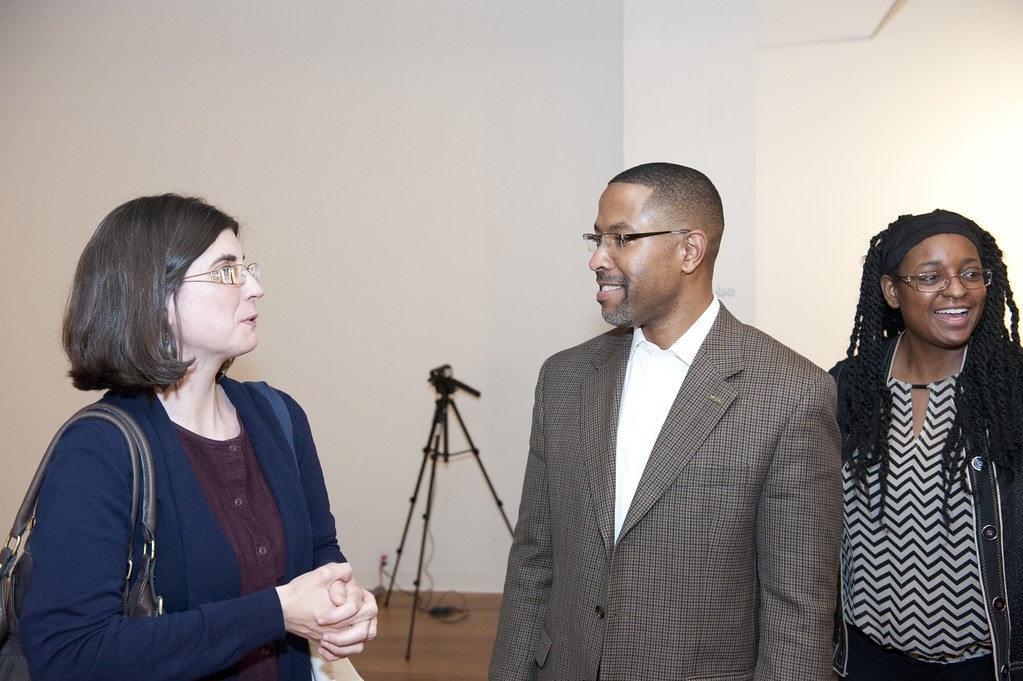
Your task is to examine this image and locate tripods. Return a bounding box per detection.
[382,364,515,661]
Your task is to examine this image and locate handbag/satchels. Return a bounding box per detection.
[0,403,159,681]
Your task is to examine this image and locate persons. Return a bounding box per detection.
[488,162,845,681]
[15,193,379,681]
[827,209,1023,681]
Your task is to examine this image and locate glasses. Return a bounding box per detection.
[181,261,261,286]
[889,267,994,292]
[582,230,691,255]
